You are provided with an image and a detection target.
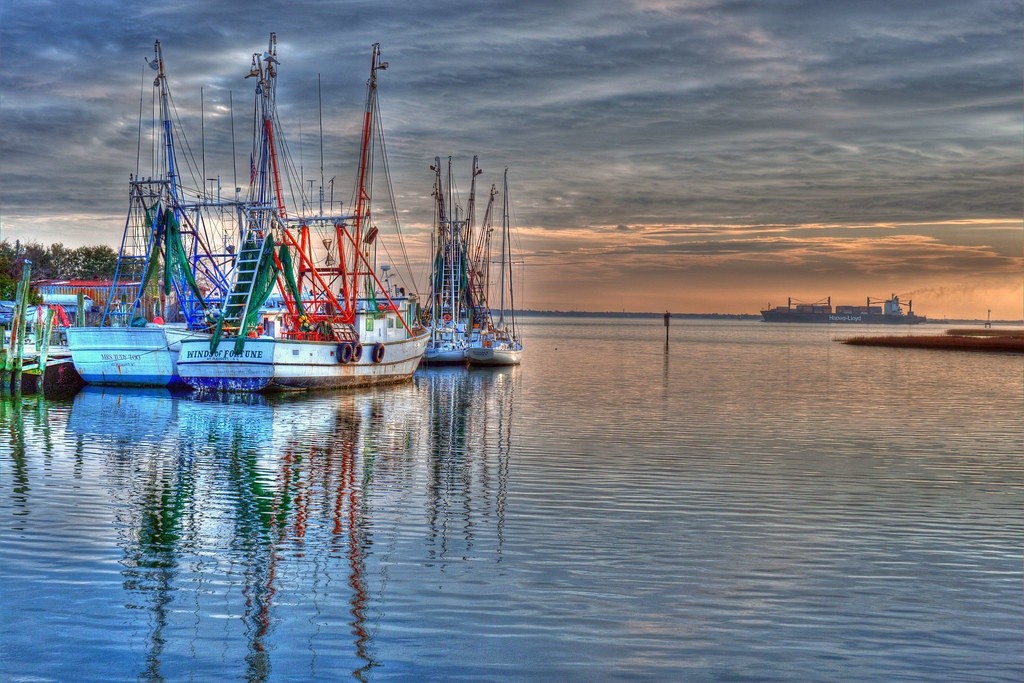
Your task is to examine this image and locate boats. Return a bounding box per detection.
[759,292,927,325]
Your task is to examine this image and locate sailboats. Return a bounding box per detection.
[0,30,523,399]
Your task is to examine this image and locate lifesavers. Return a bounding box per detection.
[444,314,451,321]
[336,343,354,363]
[372,343,385,362]
[354,342,362,361]
